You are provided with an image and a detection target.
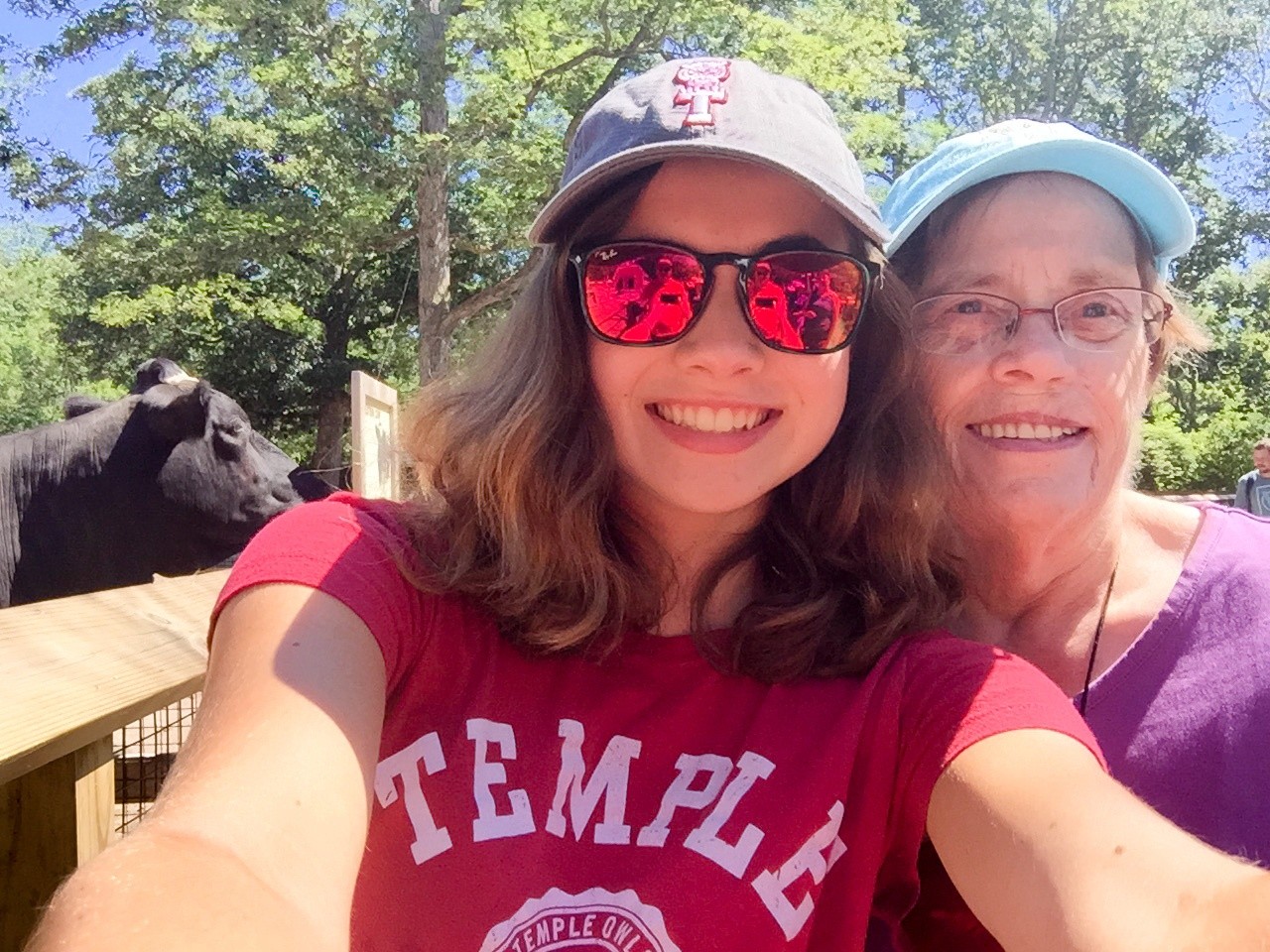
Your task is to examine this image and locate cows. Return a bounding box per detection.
[0,355,342,609]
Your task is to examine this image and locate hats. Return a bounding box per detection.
[880,118,1197,284]
[526,56,892,248]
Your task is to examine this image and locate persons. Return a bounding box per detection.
[876,116,1269,869]
[27,56,1267,951]
[1229,447,1267,519]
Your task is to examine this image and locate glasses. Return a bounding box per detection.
[566,235,880,356]
[909,287,1173,357]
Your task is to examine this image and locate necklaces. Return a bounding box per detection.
[1074,556,1121,720]
